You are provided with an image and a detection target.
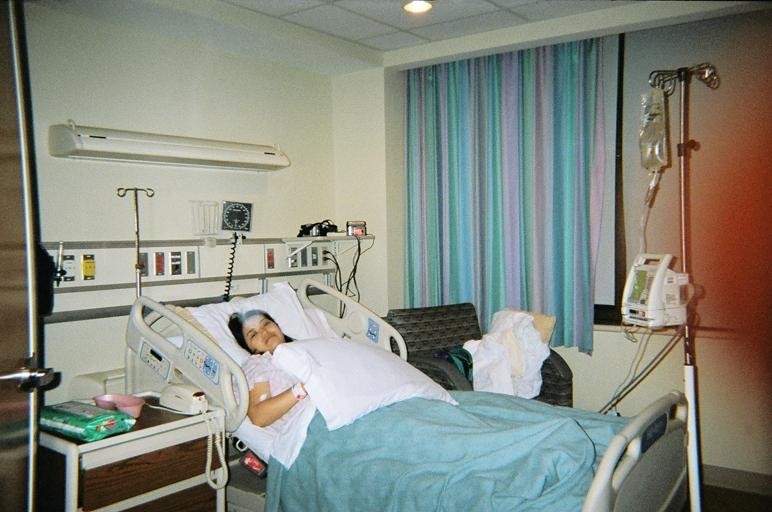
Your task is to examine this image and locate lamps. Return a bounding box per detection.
[49,123,291,173]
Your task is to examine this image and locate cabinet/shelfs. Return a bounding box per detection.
[37,389,228,512]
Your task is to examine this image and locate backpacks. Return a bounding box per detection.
[434,348,473,391]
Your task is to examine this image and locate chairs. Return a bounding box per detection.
[382,302,577,409]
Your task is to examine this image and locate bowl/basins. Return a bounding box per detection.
[91,394,145,419]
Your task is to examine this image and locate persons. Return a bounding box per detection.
[226,308,309,428]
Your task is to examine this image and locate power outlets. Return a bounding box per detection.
[320,246,329,266]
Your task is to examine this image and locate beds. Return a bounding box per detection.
[124,277,691,512]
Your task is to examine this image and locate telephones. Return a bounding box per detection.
[158,383,209,416]
